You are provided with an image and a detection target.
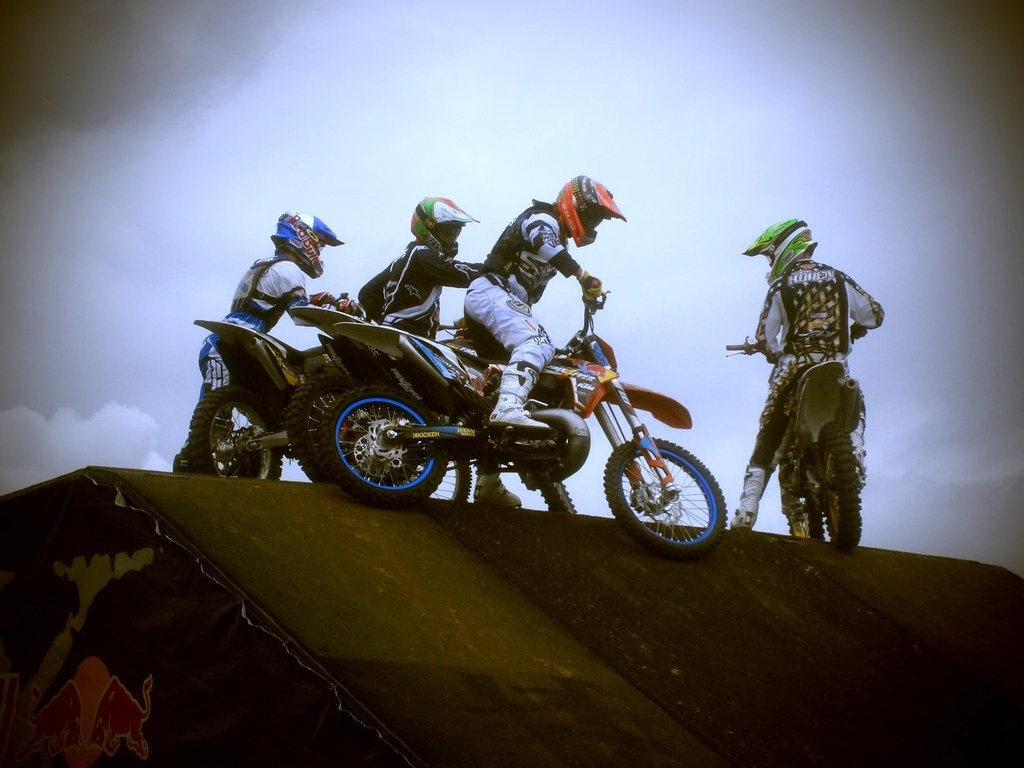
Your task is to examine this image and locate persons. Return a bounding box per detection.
[358,197,488,341]
[731,221,884,530]
[173,209,357,476]
[465,175,625,509]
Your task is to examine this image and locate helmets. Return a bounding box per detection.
[742,219,819,286]
[558,176,628,247]
[270,211,345,279]
[410,196,480,258]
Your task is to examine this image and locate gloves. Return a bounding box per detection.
[311,292,335,306]
[850,322,868,344]
[336,297,354,314]
[578,271,601,301]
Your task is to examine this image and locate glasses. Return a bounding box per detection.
[436,226,461,239]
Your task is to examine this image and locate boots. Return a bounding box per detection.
[488,361,551,440]
[474,474,521,507]
[731,466,773,530]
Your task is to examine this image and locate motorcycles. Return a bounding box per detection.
[725,337,867,551]
[185,277,728,561]
